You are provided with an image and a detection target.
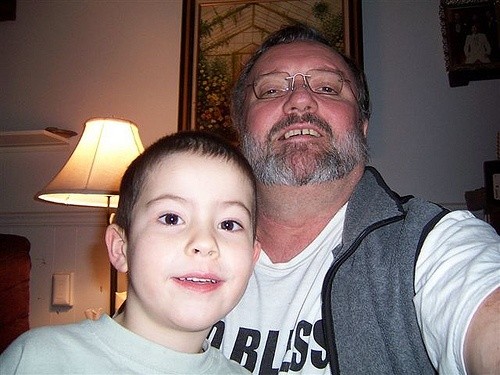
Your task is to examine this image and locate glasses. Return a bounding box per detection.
[238,68,362,111]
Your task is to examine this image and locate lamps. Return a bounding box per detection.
[35,118,146,318]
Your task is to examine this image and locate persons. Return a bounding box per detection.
[0,128,262,375]
[206,23,500,375]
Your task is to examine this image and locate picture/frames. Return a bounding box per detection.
[439,0,500,88]
[177,0,364,155]
